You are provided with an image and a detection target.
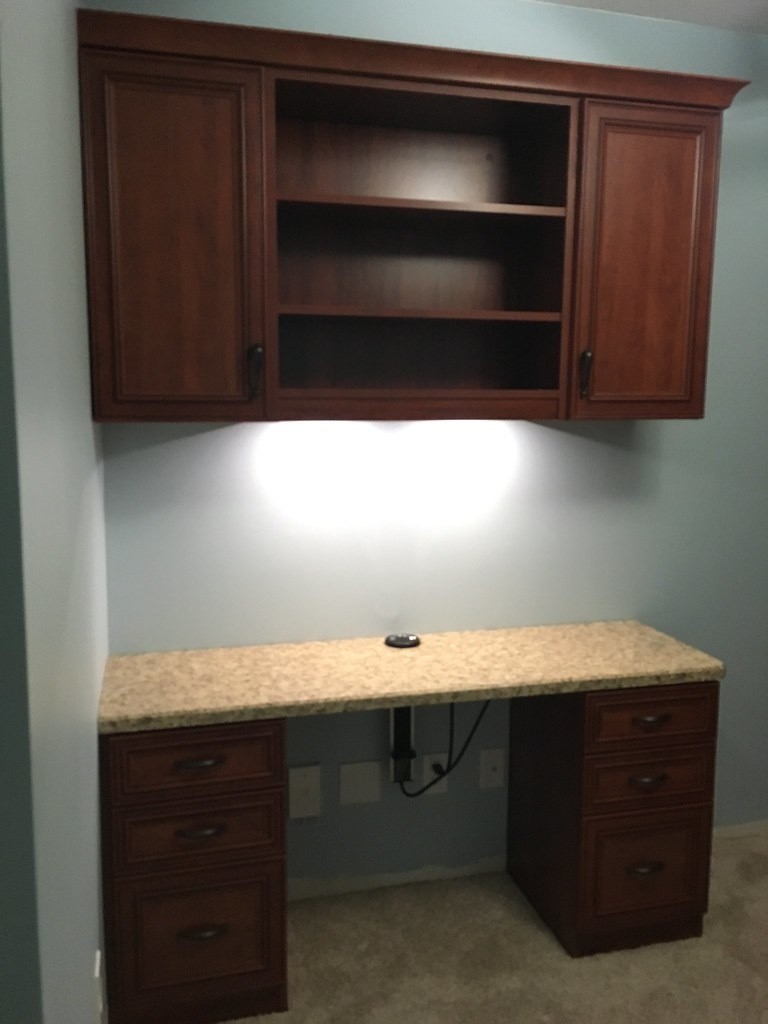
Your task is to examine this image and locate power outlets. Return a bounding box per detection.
[482,747,503,789]
[423,752,449,792]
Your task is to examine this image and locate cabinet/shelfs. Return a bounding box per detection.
[96,718,293,1024]
[76,6,754,424]
[506,679,720,959]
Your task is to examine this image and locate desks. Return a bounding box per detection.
[96,620,725,740]
